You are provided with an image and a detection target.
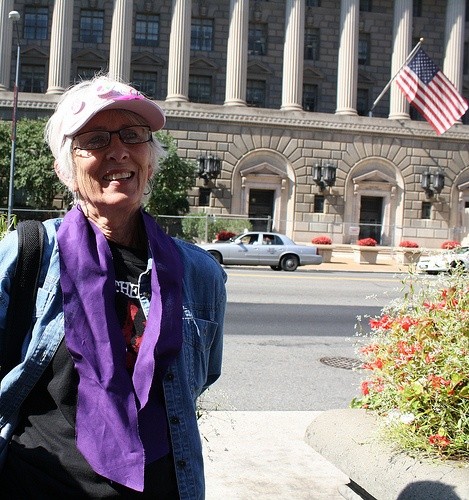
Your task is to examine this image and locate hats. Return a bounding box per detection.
[53,77,167,137]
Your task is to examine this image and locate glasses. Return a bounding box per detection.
[71,125,154,151]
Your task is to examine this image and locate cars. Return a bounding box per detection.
[193,231,322,272]
[417,246,469,277]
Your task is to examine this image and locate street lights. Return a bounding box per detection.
[6,10,21,233]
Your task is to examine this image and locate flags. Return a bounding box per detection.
[394,38,469,136]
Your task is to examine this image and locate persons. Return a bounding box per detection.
[0,74,227,500]
[265,237,272,245]
[249,236,257,245]
